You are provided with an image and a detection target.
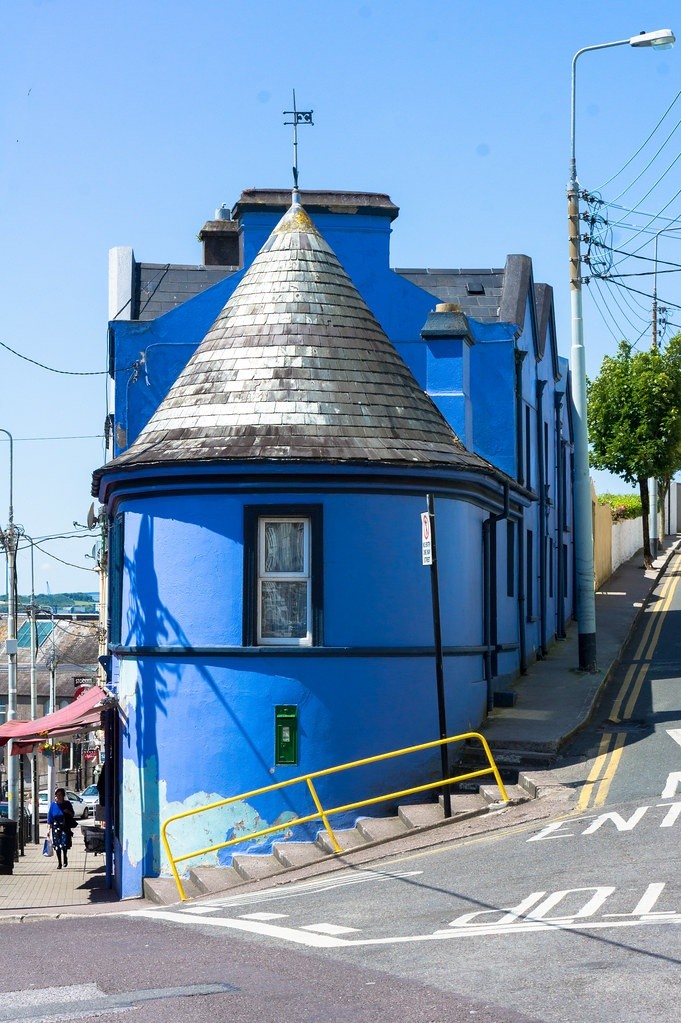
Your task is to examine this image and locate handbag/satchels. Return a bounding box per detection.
[67,802,78,828]
[43,834,53,856]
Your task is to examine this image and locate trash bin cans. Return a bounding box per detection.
[0,816,18,875]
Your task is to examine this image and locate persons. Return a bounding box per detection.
[47,788,75,870]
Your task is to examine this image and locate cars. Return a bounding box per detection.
[29,784,100,818]
[0,802,31,822]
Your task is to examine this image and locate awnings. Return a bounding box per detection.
[0,685,116,756]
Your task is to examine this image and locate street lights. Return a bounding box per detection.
[564,25,677,674]
[0,600,54,811]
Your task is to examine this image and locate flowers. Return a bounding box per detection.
[33,730,49,739]
[39,742,69,757]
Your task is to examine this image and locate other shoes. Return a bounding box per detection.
[58,864,62,869]
[63,858,67,867]
